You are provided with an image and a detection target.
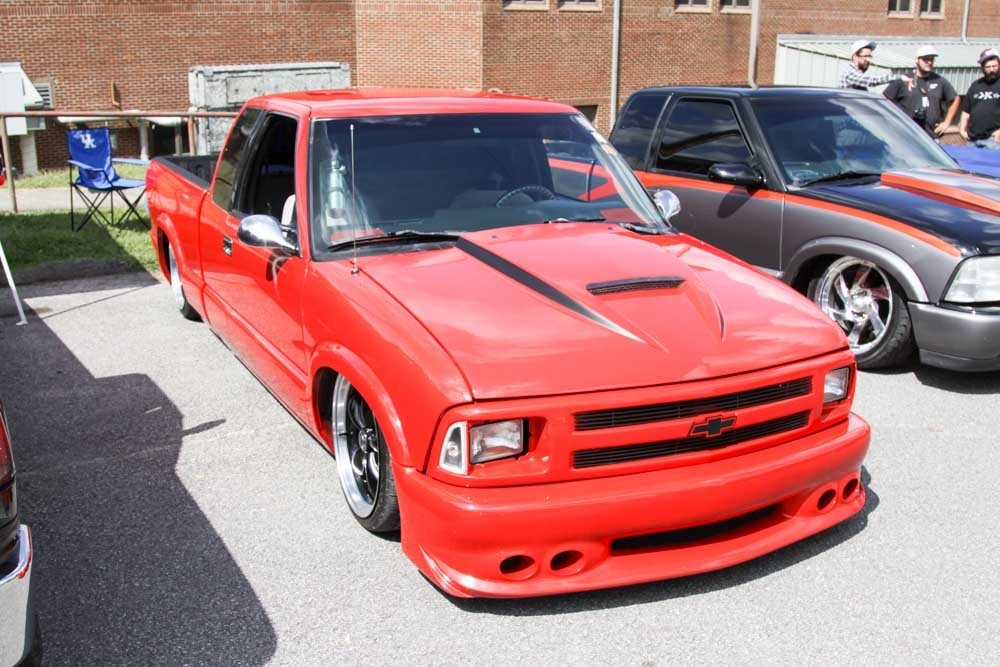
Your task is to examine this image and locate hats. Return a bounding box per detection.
[979,48,999,65]
[851,39,876,57]
[917,45,938,57]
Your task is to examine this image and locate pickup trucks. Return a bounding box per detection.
[545,85,1000,372]
[145,87,871,601]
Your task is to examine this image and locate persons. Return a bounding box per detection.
[960,49,1000,151]
[837,40,914,160]
[883,47,960,142]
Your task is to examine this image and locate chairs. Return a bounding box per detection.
[65,126,151,239]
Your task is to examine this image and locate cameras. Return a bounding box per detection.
[912,108,926,123]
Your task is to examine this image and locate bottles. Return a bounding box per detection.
[325,161,346,209]
